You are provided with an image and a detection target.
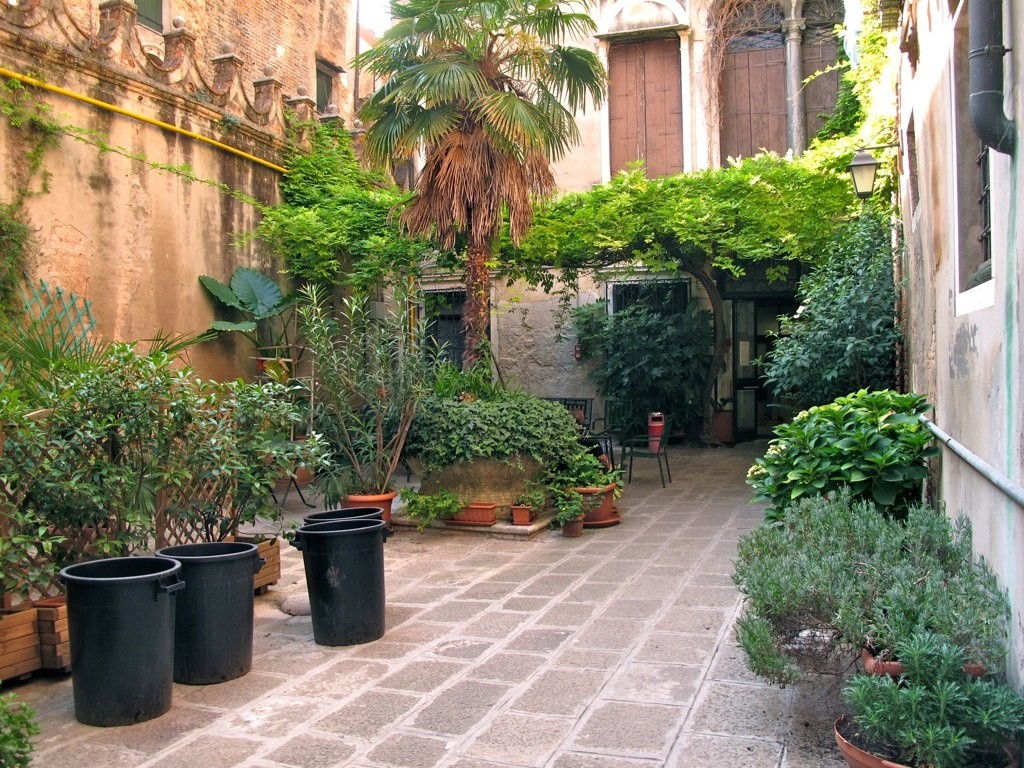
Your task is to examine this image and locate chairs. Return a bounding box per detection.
[591,399,632,459]
[618,412,674,489]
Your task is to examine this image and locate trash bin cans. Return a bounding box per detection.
[59,556,186,727]
[303,507,384,524]
[154,542,266,686]
[648,413,664,458]
[289,519,394,645]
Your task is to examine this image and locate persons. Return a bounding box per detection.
[548,407,622,502]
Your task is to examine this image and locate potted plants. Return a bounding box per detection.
[710,397,734,444]
[294,277,451,530]
[560,499,586,537]
[570,456,621,529]
[509,495,539,526]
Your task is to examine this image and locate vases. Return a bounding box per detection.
[216,535,281,596]
[257,435,316,487]
[0,594,74,686]
[452,502,497,523]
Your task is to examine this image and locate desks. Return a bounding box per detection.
[237,439,316,516]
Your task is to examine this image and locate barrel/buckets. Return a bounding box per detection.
[60,556,189,726]
[155,542,268,686]
[289,507,394,646]
